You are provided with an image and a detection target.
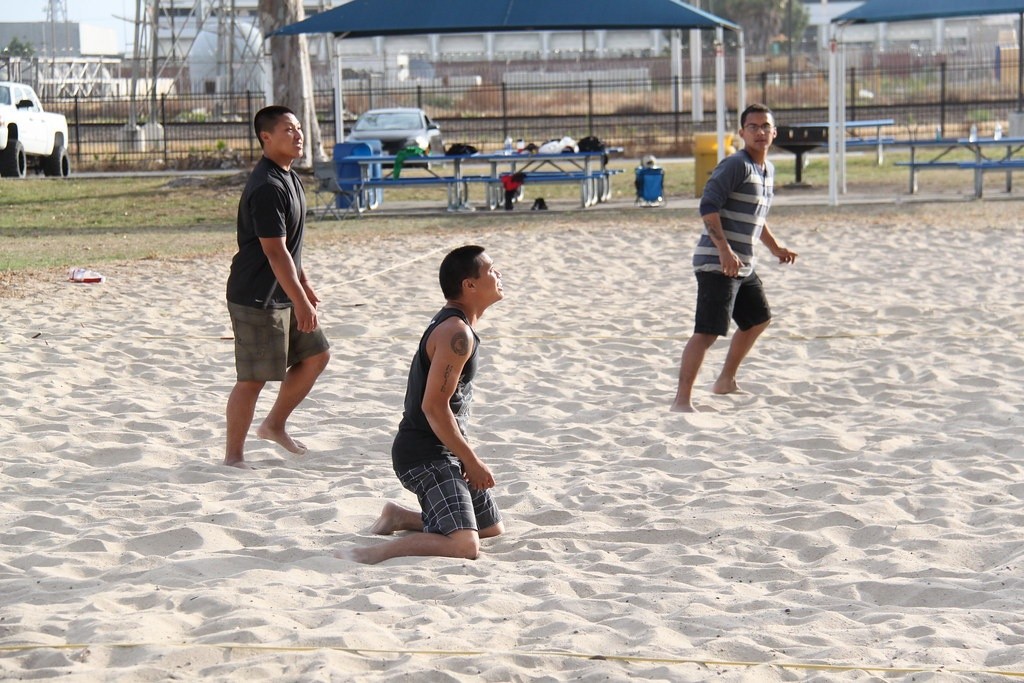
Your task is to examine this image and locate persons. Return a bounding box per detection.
[669,102,798,413]
[336,245,505,564]
[225,106,330,464]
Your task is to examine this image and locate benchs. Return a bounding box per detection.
[340,169,624,186]
[821,136,895,149]
[894,159,1024,199]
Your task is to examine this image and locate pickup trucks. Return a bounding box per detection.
[0,81,71,179]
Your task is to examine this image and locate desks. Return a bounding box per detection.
[346,151,609,214]
[772,119,896,189]
[363,146,625,211]
[893,137,1024,199]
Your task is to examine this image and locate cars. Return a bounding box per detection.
[343,106,442,169]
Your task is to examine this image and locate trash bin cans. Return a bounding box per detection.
[692,132,738,198]
[332,140,382,209]
[635,168,664,203]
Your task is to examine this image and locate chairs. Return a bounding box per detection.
[310,160,362,222]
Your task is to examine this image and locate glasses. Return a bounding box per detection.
[742,124,776,132]
